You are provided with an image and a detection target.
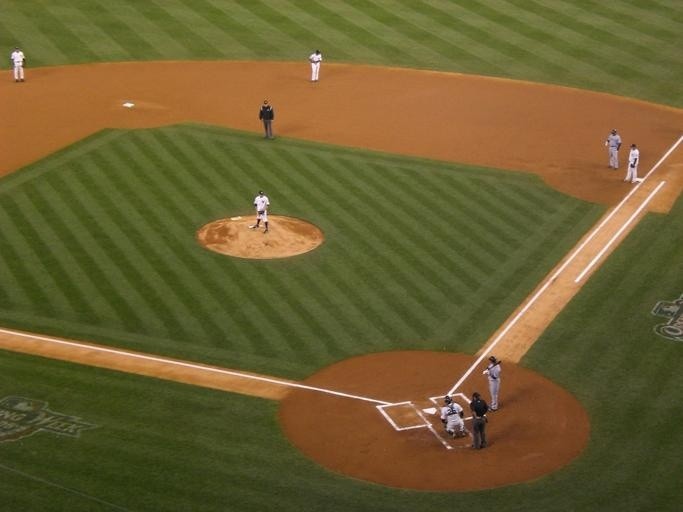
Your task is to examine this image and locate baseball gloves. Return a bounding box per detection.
[630,164,634,168]
[257,211,264,215]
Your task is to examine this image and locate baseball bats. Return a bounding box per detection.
[487,360,501,371]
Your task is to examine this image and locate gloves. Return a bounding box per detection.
[482,369,490,375]
[604,141,608,146]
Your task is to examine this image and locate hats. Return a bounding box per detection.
[263,100,268,104]
[472,392,480,397]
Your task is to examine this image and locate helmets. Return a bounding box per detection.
[258,190,263,196]
[611,128,616,133]
[488,356,496,362]
[443,395,450,401]
[315,50,320,53]
[629,144,636,148]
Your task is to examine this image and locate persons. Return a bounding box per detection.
[470,392,489,449]
[10,46,27,82]
[252,190,270,232]
[483,357,502,413]
[260,100,274,139]
[441,396,465,439]
[624,143,639,184]
[309,48,323,81]
[605,129,622,170]
